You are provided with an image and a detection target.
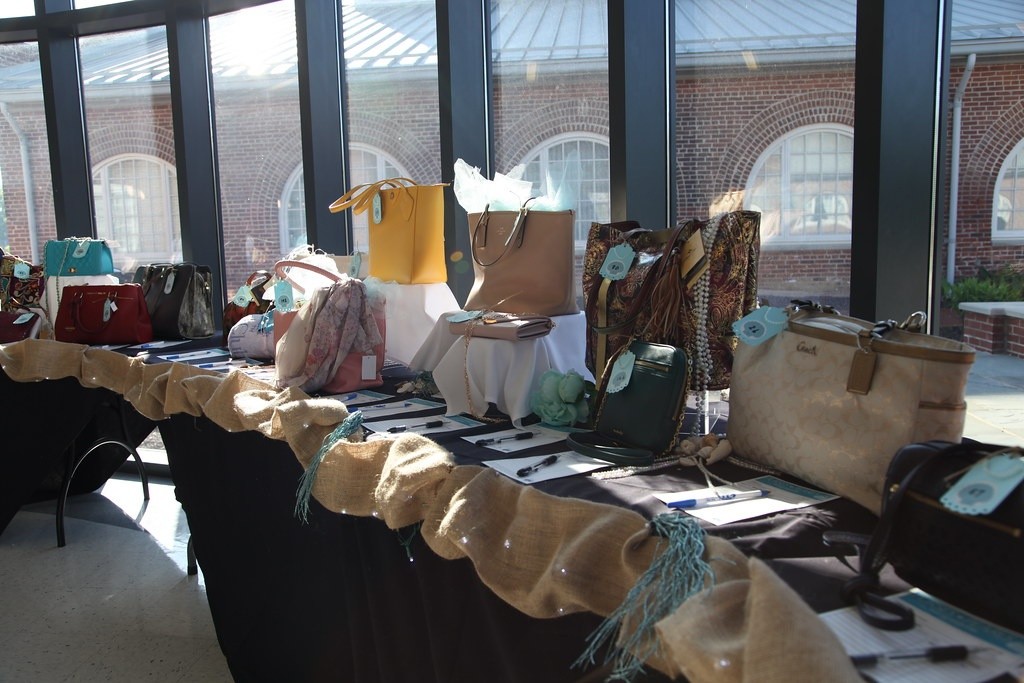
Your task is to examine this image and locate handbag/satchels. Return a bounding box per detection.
[446,198,762,465]
[725,299,1024,638]
[221,177,450,395]
[0,235,213,345]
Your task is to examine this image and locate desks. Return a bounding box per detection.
[0,329,1024,683]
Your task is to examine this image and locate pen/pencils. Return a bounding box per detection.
[849,643,996,667]
[476,432,542,446]
[166,351,211,359]
[515,453,562,478]
[387,420,452,433]
[199,359,252,367]
[668,488,771,508]
[141,341,167,348]
[348,403,413,413]
[340,393,357,402]
